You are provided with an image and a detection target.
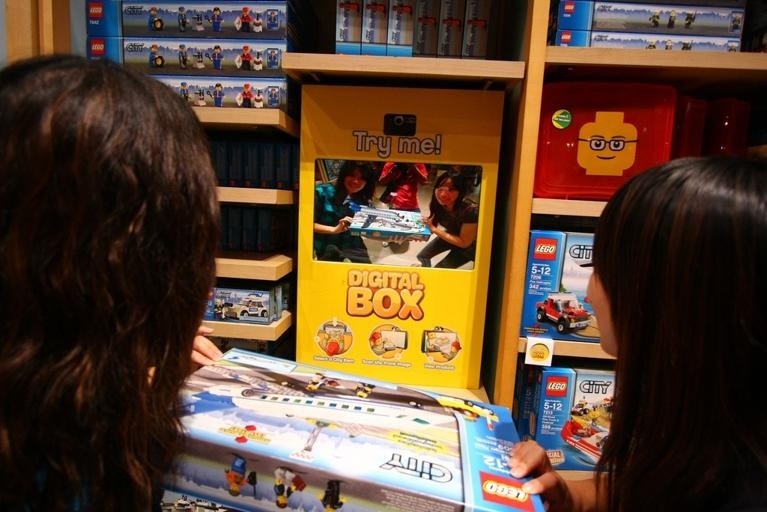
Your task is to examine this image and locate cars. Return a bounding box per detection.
[535,289,592,336]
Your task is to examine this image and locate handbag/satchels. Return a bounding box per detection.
[380,181,398,204]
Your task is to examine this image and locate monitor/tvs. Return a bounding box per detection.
[312,158,483,271]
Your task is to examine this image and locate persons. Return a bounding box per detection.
[315,161,378,265]
[416,168,482,268]
[504,149,767,512]
[378,162,429,214]
[0,50,224,511]
